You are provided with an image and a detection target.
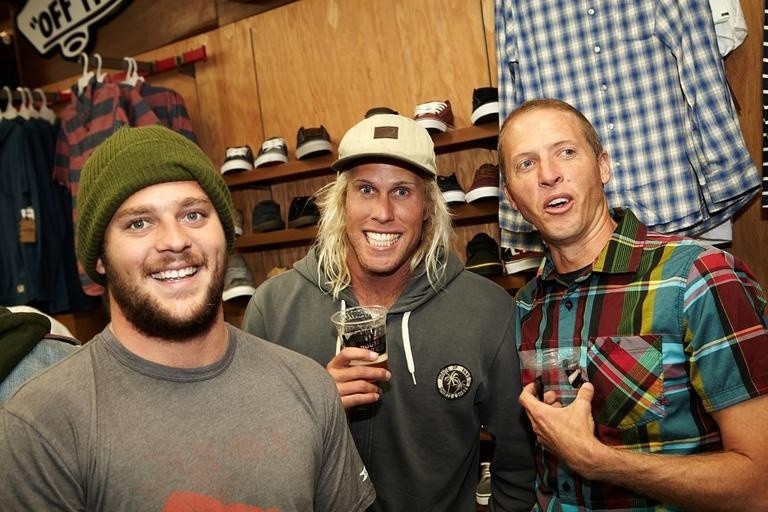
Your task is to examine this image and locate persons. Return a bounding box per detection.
[240,112,537,512]
[0,122,378,512]
[496,98,768,512]
[0,303,82,401]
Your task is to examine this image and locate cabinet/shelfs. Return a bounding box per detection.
[221,120,530,317]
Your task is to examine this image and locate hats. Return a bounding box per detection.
[77,126,235,283]
[331,114,438,183]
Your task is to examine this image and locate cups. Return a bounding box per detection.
[331,305,389,382]
[527,346,582,407]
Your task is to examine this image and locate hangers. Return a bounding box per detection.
[1,50,185,142]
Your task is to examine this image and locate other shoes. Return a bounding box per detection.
[501,247,545,275]
[235,210,244,236]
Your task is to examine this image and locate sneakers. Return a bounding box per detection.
[288,196,319,227]
[476,462,491,505]
[222,255,256,301]
[471,88,500,127]
[436,173,466,203]
[464,163,500,203]
[221,146,253,176]
[252,201,285,233]
[414,100,455,133]
[255,137,287,169]
[464,232,504,276]
[295,124,332,161]
